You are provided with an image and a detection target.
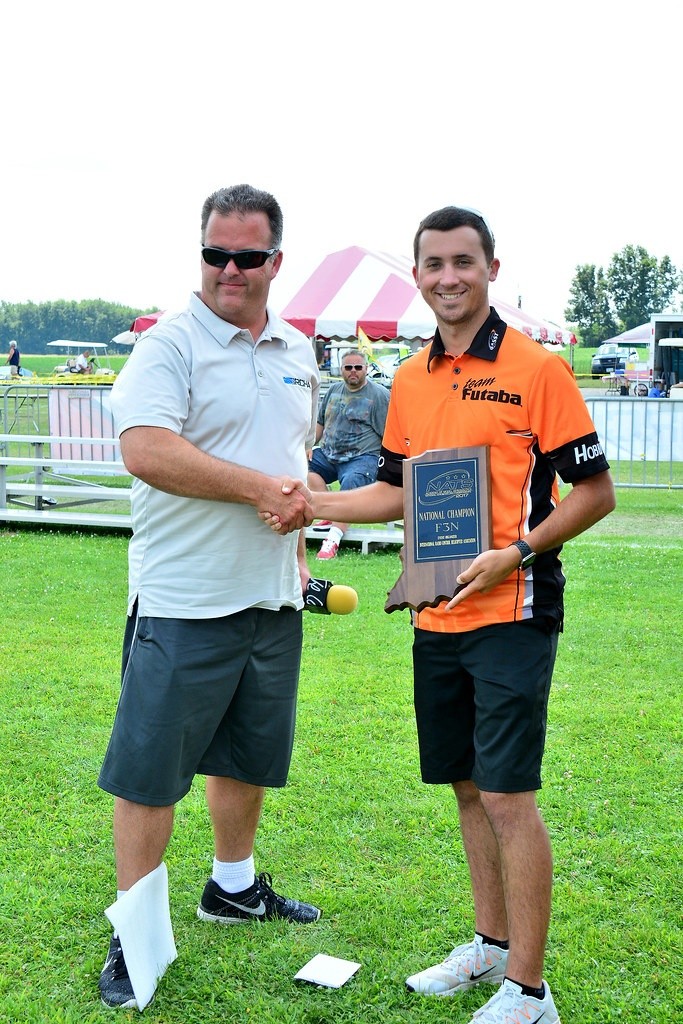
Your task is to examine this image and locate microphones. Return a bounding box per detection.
[302,578,358,615]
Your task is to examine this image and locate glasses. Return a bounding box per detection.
[342,364,366,371]
[201,244,278,269]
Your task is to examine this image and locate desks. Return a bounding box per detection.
[603,375,624,396]
[0,394,48,434]
[628,379,664,398]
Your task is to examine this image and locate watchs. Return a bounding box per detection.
[507,539,537,571]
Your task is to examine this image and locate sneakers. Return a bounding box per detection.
[469,977,563,1024]
[315,538,339,560]
[197,873,322,925]
[99,933,155,1009]
[312,519,333,529]
[404,934,509,996]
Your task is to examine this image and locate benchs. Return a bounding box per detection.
[0,434,404,556]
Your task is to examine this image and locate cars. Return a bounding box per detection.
[370,353,414,378]
[590,342,639,378]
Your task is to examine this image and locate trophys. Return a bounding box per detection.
[384,444,492,614]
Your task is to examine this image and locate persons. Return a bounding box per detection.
[5,340,20,380]
[305,350,390,561]
[98,185,313,1009]
[75,349,94,375]
[257,205,617,1024]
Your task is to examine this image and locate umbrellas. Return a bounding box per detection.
[112,330,139,346]
[602,321,651,355]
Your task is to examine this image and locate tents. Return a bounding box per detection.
[131,238,576,398]
